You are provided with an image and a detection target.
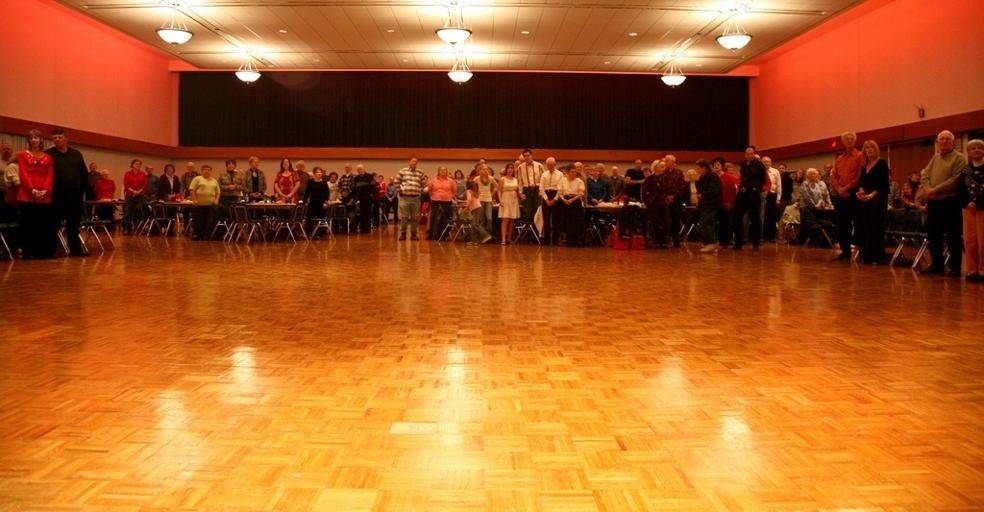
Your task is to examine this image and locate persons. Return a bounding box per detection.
[1,130,396,284]
[394,129,983,282]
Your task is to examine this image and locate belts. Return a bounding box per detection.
[400,194,419,197]
[545,189,558,192]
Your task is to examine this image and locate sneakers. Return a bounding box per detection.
[399,232,407,240]
[834,249,888,266]
[700,242,760,253]
[947,270,962,279]
[69,249,90,257]
[22,249,59,260]
[410,232,420,240]
[543,240,585,248]
[481,235,492,244]
[965,273,983,283]
[920,264,945,276]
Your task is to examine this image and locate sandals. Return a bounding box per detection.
[500,239,515,246]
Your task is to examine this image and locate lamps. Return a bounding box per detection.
[435,2,472,45]
[447,42,473,85]
[661,56,687,89]
[156,3,194,46]
[716,8,753,51]
[235,50,261,84]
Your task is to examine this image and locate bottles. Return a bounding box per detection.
[238,190,279,203]
